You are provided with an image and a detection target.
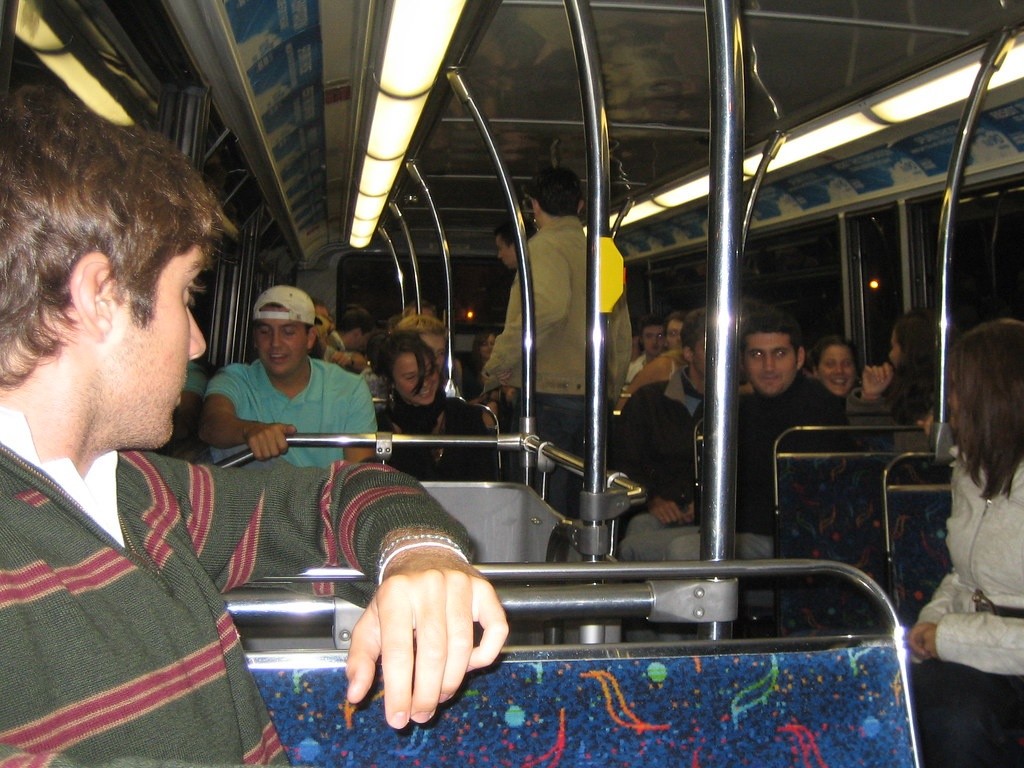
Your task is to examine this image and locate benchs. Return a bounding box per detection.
[203,365,958,768]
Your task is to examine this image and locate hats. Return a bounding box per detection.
[254,284,315,325]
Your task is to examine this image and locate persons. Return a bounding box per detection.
[609,305,850,643]
[907,319,1024,768]
[0,85,510,768]
[805,334,863,408]
[846,310,960,456]
[367,330,502,483]
[308,299,501,429]
[460,166,631,520]
[199,285,378,468]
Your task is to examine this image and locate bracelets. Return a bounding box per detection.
[378,542,470,586]
[378,534,462,573]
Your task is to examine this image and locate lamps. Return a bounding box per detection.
[10,0,1024,252]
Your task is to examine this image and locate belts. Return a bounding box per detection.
[976,601,1024,620]
